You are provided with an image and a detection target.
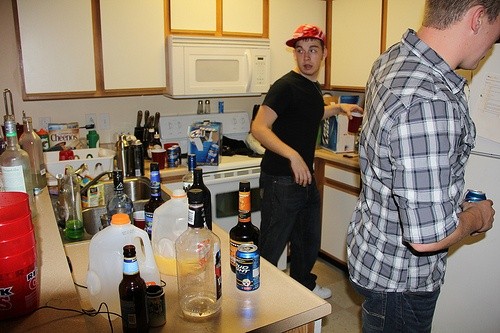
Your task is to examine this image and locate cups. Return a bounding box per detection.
[151,149,167,169]
[0,191,41,317]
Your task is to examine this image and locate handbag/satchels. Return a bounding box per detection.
[219,136,254,156]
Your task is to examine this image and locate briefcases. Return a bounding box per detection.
[189,119,222,166]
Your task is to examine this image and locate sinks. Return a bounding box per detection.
[103,179,151,204]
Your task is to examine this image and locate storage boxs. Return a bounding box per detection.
[43,148,117,186]
[80,176,105,210]
[320,112,356,154]
[188,120,223,167]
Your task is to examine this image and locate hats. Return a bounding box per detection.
[286,24,324,47]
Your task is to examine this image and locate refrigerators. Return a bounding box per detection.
[430,42,500,333]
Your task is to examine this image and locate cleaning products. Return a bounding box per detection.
[62,165,84,239]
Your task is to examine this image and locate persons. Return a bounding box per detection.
[249,22,364,301]
[345,0,500,333]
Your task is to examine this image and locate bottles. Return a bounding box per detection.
[218,101,224,114]
[36,128,50,152]
[190,168,212,230]
[60,166,85,242]
[19,116,48,193]
[86,130,100,148]
[175,188,222,322]
[85,213,162,321]
[152,189,205,293]
[144,162,165,241]
[205,100,210,114]
[182,154,197,188]
[151,130,162,149]
[197,99,203,115]
[0,113,37,218]
[118,244,151,333]
[229,180,262,274]
[108,169,135,225]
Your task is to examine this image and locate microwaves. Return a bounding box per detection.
[164,34,271,99]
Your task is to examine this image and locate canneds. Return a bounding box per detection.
[462,189,488,203]
[205,144,219,163]
[218,101,224,113]
[146,285,166,327]
[167,145,182,168]
[235,244,261,292]
[188,154,197,171]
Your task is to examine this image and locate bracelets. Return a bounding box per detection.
[336,104,342,114]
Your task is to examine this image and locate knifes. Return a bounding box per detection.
[136,110,160,142]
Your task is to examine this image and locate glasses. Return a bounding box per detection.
[293,48,322,55]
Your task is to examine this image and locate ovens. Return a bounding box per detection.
[182,173,288,271]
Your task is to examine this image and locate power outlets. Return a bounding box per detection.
[39,117,51,130]
[85,113,97,126]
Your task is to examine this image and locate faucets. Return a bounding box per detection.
[80,172,112,195]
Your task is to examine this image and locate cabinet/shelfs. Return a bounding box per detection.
[267,0,327,88]
[327,0,425,93]
[319,158,362,273]
[12,0,170,101]
[169,0,269,39]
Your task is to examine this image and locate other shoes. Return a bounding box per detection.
[312,283,332,300]
